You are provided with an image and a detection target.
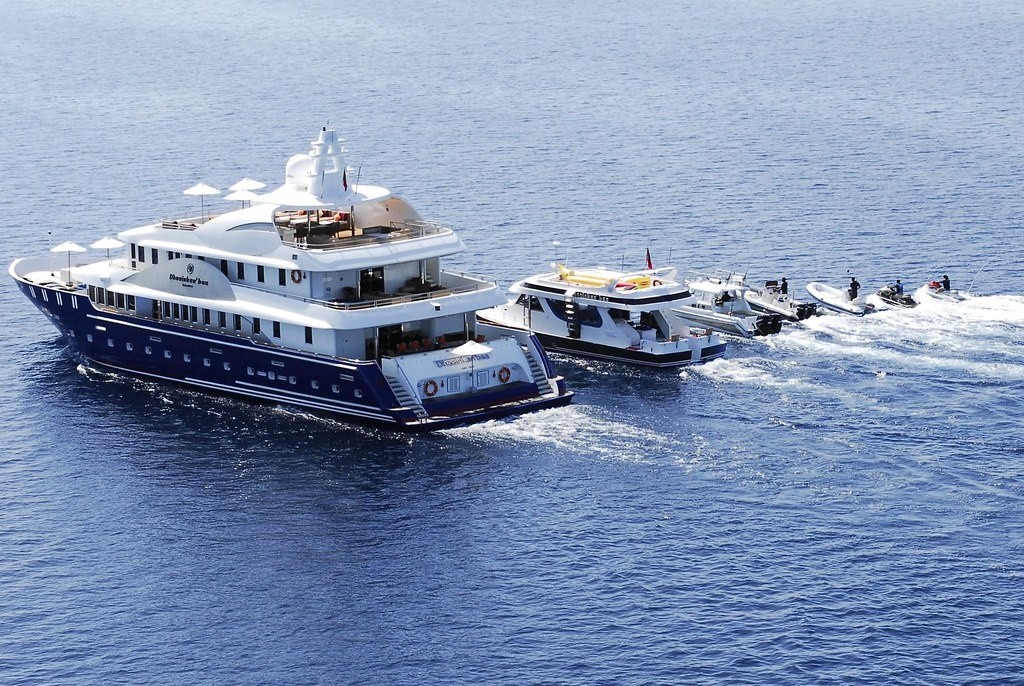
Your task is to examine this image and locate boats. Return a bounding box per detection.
[806,281,876,317]
[475,242,728,369]
[670,272,784,338]
[719,272,818,323]
[7,118,576,433]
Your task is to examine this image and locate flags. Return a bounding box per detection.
[647,249,652,269]
[343,170,347,191]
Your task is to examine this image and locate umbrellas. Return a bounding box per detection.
[183,182,220,224]
[91,236,125,259]
[224,177,266,209]
[451,340,493,387]
[51,241,86,285]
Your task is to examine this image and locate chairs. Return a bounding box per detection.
[275,210,351,232]
[294,228,339,245]
[396,335,446,353]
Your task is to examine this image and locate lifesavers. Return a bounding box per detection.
[498,367,511,382]
[424,380,438,396]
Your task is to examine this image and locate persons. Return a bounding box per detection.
[934,275,950,294]
[850,277,860,301]
[717,291,731,305]
[776,278,788,299]
[889,280,903,298]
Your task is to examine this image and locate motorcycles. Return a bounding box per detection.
[877,285,918,309]
[926,280,967,304]
